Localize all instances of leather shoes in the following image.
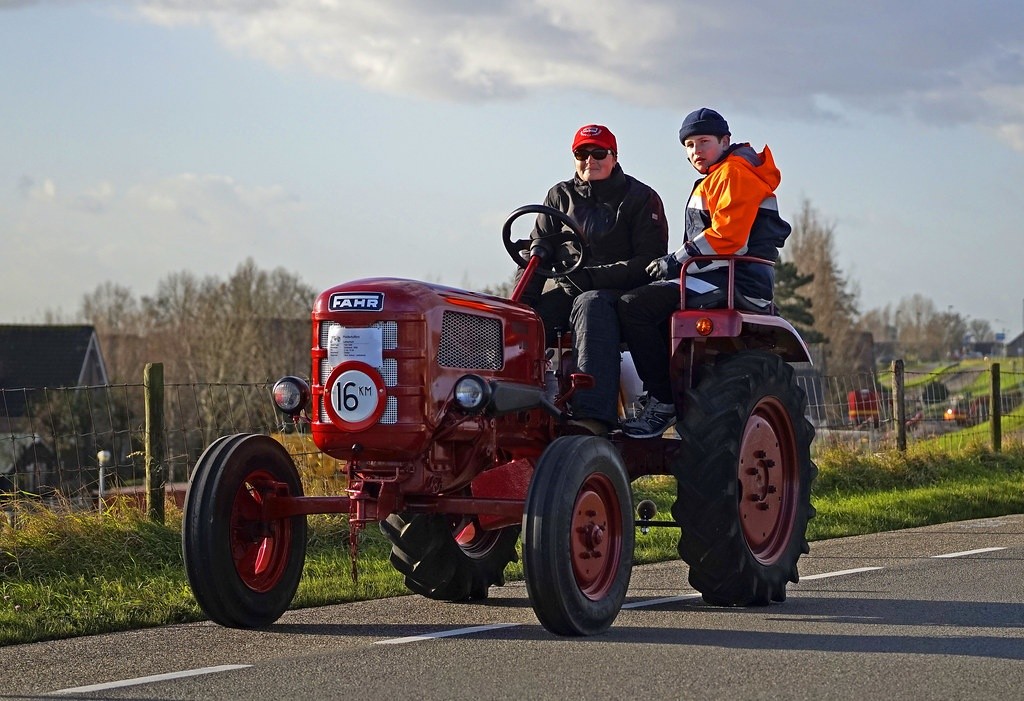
[569,418,609,438]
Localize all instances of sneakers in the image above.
[621,396,677,439]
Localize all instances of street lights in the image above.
[95,449,112,514]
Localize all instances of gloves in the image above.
[644,254,679,281]
[556,261,588,297]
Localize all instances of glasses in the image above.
[574,148,611,160]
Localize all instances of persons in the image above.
[619,107,793,438]
[516,121,668,438]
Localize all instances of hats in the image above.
[680,108,731,145]
[573,125,618,157]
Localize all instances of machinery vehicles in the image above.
[180,202,823,641]
